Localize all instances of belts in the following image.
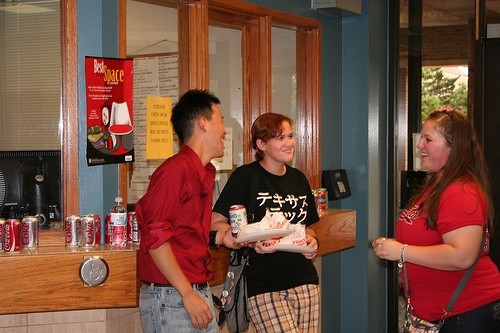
[141,282,207,291]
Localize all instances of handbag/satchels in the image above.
[403,312,440,333]
[217,250,250,333]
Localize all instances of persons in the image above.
[372,110,500,333]
[210,112,321,333]
[135,88,226,333]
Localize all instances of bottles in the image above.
[110,197,127,248]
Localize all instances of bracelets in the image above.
[311,235,319,249]
[398,244,407,268]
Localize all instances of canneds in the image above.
[316,188,329,212]
[0,216,38,252]
[64,214,101,249]
[105,212,141,246]
[229,204,248,238]
